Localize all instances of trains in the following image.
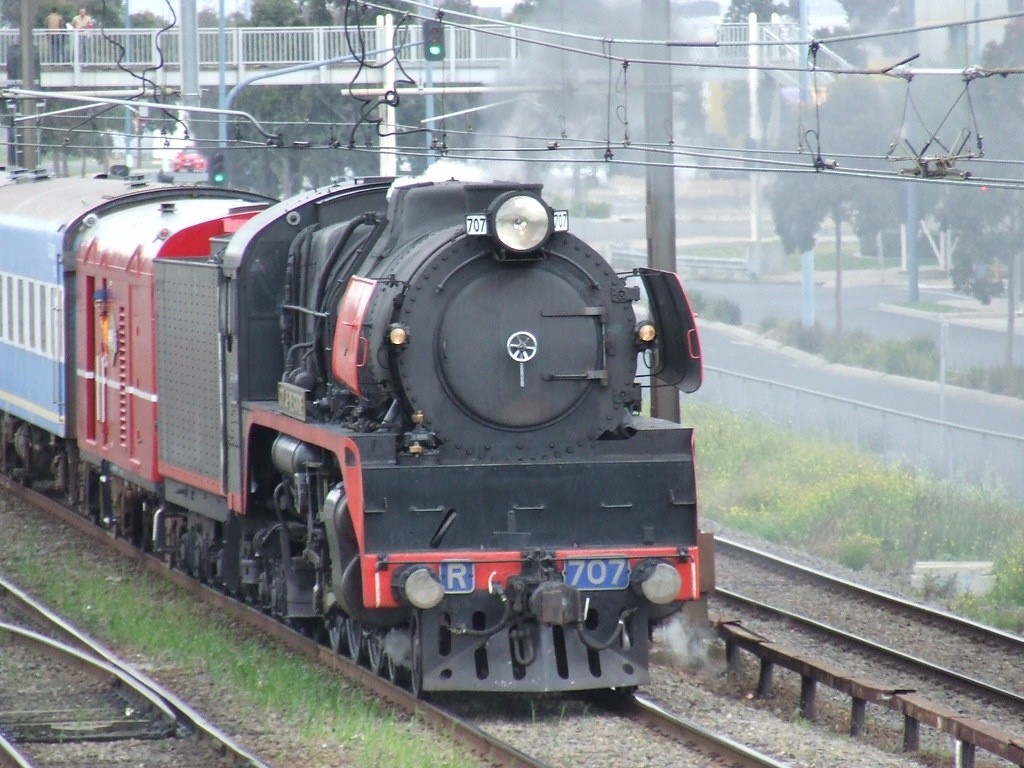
[0,163,704,716]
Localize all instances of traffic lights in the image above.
[208,150,226,186]
[421,20,446,63]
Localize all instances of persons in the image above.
[976,258,990,304]
[45,8,66,63]
[72,8,92,63]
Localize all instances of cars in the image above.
[172,144,209,173]
